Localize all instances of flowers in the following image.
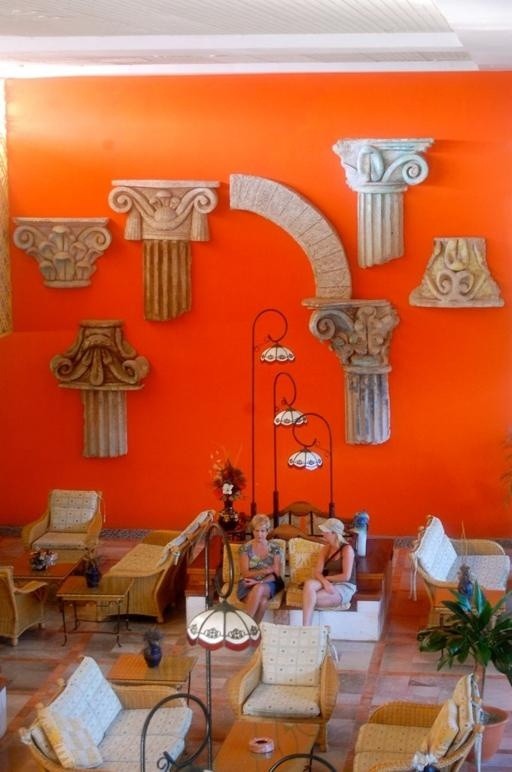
[207,445,248,501]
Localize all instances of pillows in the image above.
[35,704,104,770]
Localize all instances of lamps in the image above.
[246,308,334,526]
[186,522,263,772]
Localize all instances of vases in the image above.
[218,504,240,531]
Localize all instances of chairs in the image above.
[220,524,358,624]
[230,621,341,754]
[1,489,103,648]
[410,512,511,638]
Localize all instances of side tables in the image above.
[105,652,198,713]
[56,575,135,649]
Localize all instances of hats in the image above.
[319,517,344,535]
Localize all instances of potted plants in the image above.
[413,564,512,762]
[141,628,163,668]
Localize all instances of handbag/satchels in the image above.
[272,572,285,593]
[322,568,328,575]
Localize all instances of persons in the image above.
[302,518,358,627]
[237,514,285,624]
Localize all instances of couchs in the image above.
[97,509,216,624]
[0,652,194,772]
[352,669,486,772]
